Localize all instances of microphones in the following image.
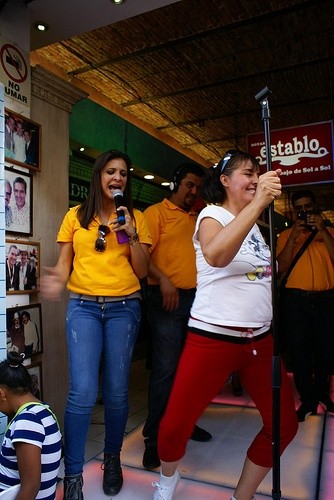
[113,189,125,225]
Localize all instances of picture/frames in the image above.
[4,104,44,423]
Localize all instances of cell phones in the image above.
[297,209,307,227]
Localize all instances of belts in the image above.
[70,292,142,302]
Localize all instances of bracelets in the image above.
[130,234,139,245]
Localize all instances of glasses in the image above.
[220,150,248,173]
[94,225,110,250]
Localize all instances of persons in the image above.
[276,189,334,423]
[0,351,61,500]
[143,163,213,470]
[11,310,38,355]
[5,177,30,232]
[5,245,37,290]
[5,115,36,165]
[39,151,153,500]
[152,150,298,500]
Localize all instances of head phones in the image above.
[169,163,203,192]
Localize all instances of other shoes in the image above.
[316,394,334,414]
[143,437,159,470]
[191,424,212,441]
[296,401,319,422]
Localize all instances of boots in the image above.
[100,452,123,495]
[63,474,84,500]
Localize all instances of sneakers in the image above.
[152,468,180,500]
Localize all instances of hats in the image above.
[12,313,20,320]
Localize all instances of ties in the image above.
[9,131,11,136]
